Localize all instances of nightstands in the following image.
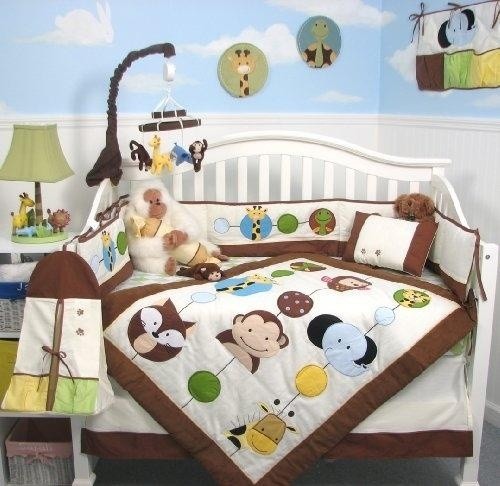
[0,233,76,264]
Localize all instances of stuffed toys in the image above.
[46,206,72,232]
[392,190,436,224]
[124,180,231,279]
[10,193,35,236]
[128,138,207,175]
[174,264,230,281]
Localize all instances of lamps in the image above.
[0,124,75,243]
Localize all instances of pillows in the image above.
[343,211,439,278]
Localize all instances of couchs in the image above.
[60,132,499,486]
